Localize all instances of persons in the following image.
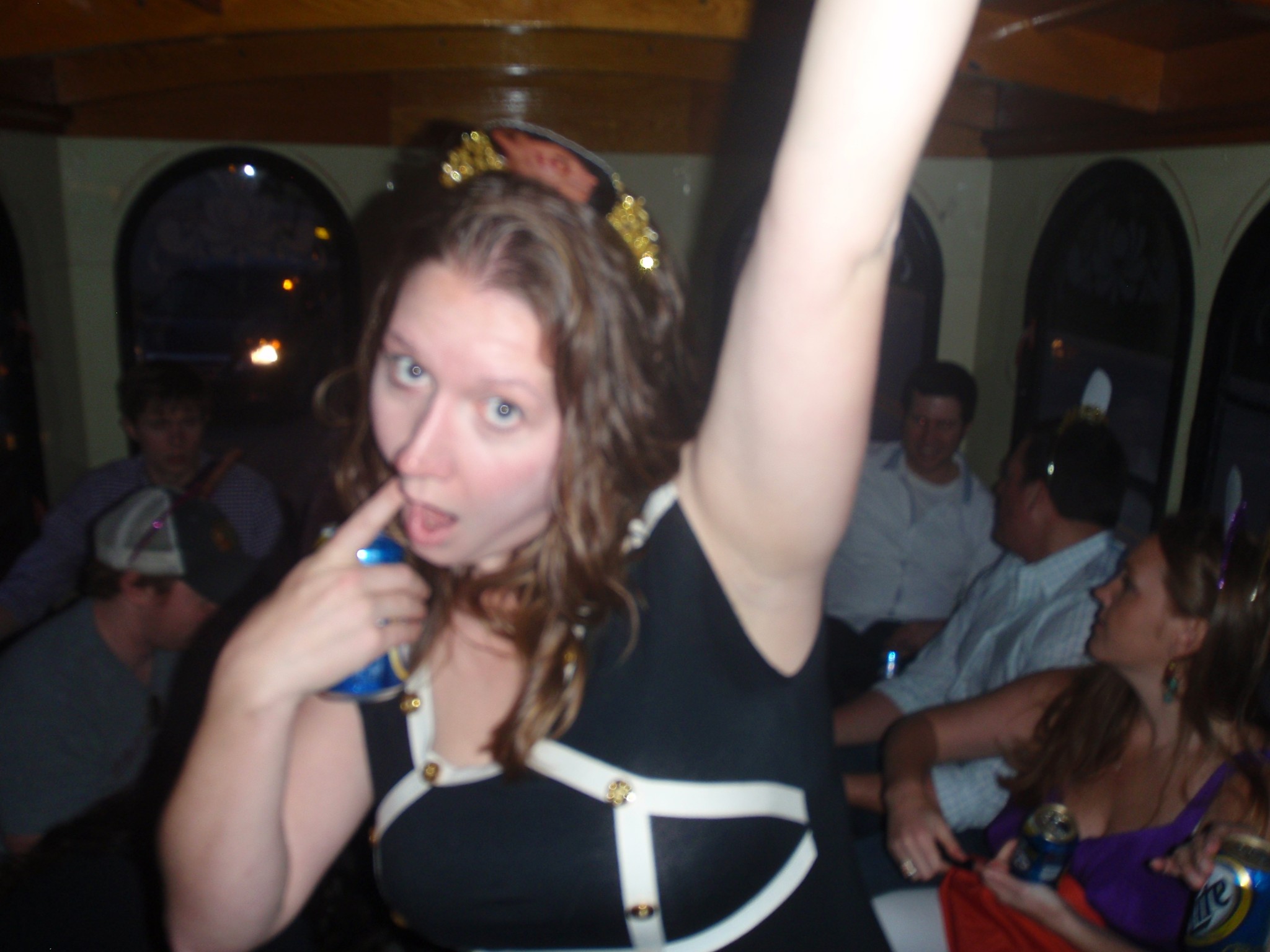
[0,363,283,952]
[825,358,1270,952]
[161,0,986,952]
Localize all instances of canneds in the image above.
[879,644,902,681]
[1178,832,1270,952]
[316,524,405,703]
[1009,803,1078,891]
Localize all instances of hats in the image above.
[92,483,255,604]
